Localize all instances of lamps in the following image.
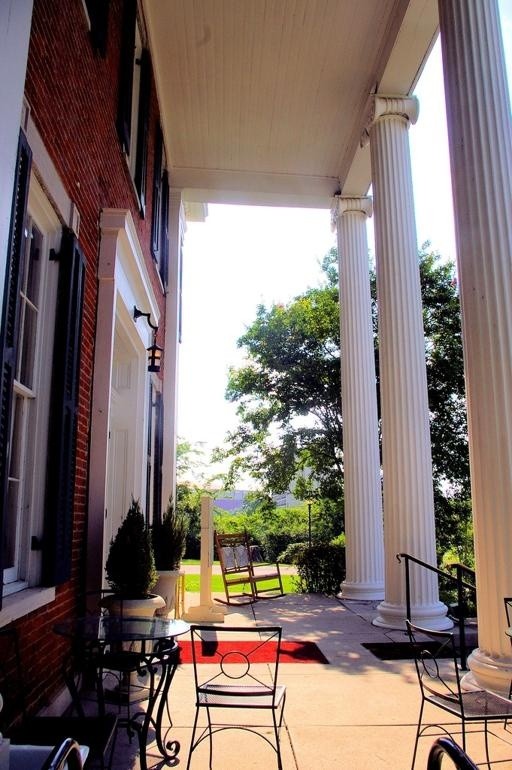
[134,305,164,372]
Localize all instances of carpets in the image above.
[360,641,459,661]
[428,634,478,657]
[168,640,330,664]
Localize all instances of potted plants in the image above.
[96,493,167,704]
[150,492,187,613]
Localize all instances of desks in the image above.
[54,615,191,770]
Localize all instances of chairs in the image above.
[80,589,174,742]
[41,737,83,770]
[213,526,286,606]
[405,619,512,770]
[0,628,118,770]
[503,597,512,730]
[427,736,479,770]
[187,625,287,770]
